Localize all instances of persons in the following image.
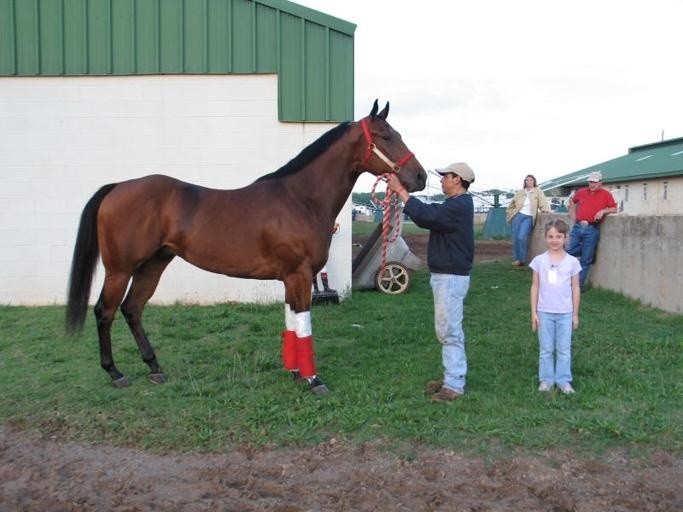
[504,174,554,267]
[561,171,618,294]
[381,161,476,404]
[526,218,583,395]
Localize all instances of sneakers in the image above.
[426,376,444,391]
[511,260,520,267]
[431,388,459,403]
[560,383,575,395]
[537,382,551,392]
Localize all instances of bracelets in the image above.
[395,187,404,198]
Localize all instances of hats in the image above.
[434,162,474,183]
[585,172,602,183]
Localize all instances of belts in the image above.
[577,220,599,227]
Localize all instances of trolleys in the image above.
[353,203,422,297]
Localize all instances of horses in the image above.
[62,99,427,397]
